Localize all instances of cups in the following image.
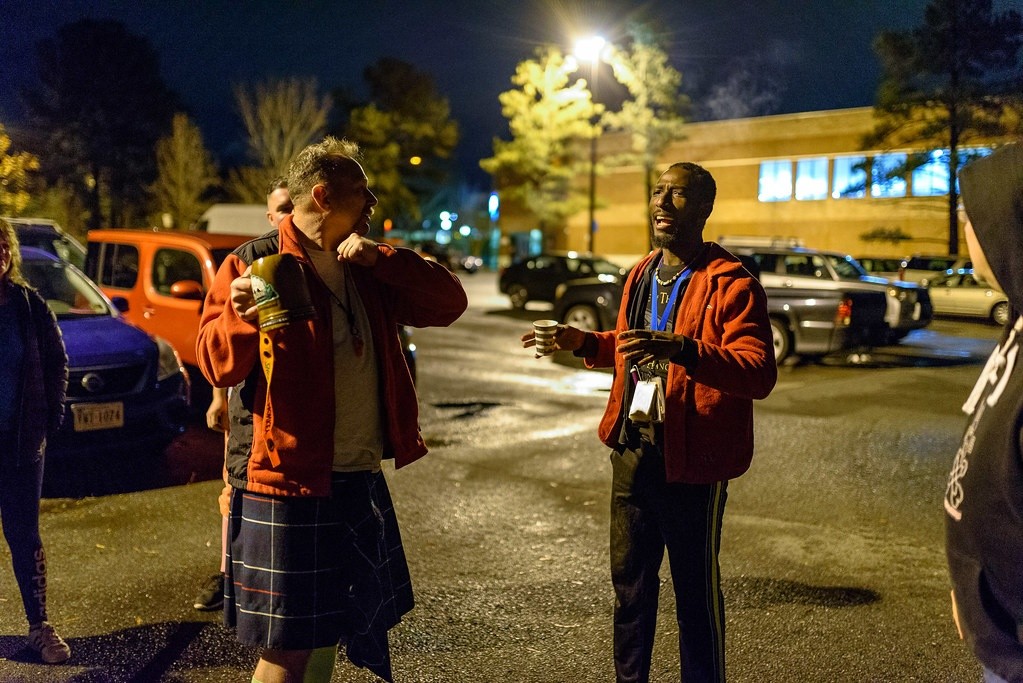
[532,319,558,356]
[250,252,316,334]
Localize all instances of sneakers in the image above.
[193,573,225,610]
[29,621,71,664]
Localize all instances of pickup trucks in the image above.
[555,243,887,365]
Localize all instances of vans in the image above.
[76,229,265,421]
[193,205,285,236]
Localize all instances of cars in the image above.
[19,248,192,473]
[920,271,1012,329]
[412,233,480,270]
[9,217,88,272]
[499,252,626,310]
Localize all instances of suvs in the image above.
[839,251,905,282]
[725,248,934,345]
[900,254,975,285]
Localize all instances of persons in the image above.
[193,138,469,683]
[0,216,71,662]
[521,161,778,683]
[944,149,1023,683]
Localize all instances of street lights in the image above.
[573,39,600,254]
